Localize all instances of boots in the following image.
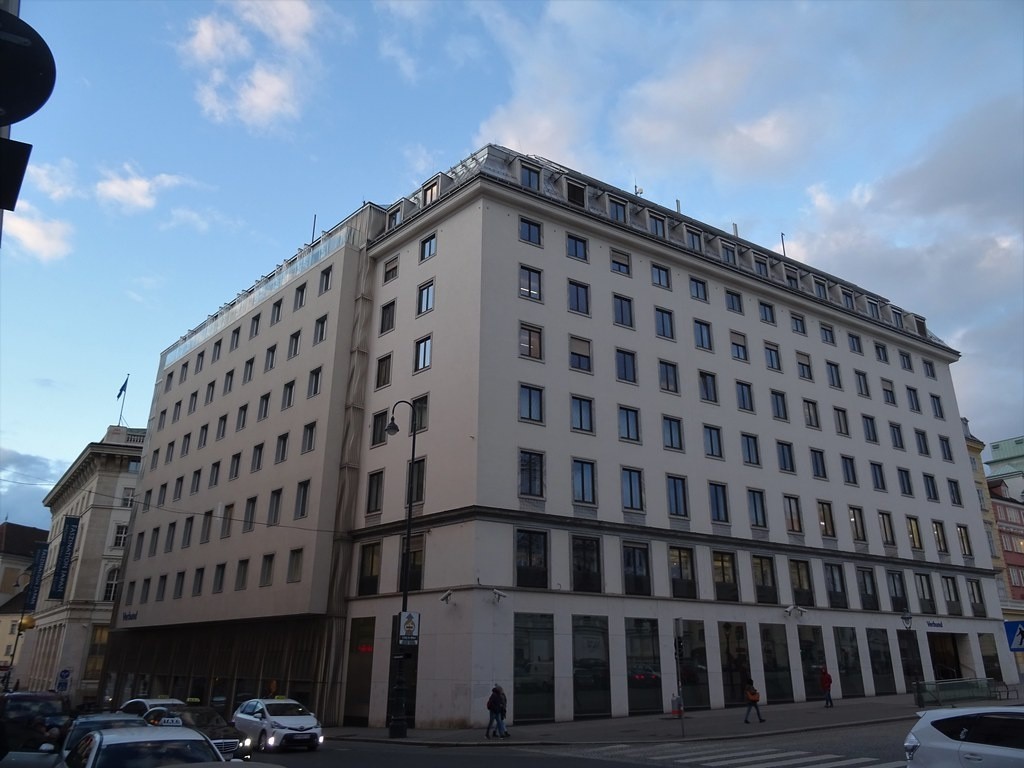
[493,731,498,737]
[504,731,510,736]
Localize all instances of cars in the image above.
[137,706,254,761]
[0,751,69,768]
[53,727,226,768]
[227,698,323,754]
[115,699,187,719]
[60,713,156,762]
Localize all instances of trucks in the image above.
[0,686,73,739]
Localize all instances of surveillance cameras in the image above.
[493,589,506,597]
[785,606,794,612]
[797,607,808,612]
[440,589,452,601]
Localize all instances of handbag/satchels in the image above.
[747,686,759,702]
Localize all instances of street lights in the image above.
[383,400,417,740]
[2,572,31,693]
[900,608,928,709]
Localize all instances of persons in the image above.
[819,666,834,708]
[485,683,511,740]
[743,679,766,725]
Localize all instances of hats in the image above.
[495,683,501,690]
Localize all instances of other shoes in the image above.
[500,735,506,738]
[760,719,766,723]
[744,720,750,724]
[485,735,490,739]
[824,704,833,708]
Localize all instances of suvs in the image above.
[902,705,1024,766]
